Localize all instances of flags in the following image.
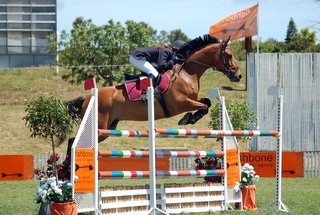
[209,3,260,43]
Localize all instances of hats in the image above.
[173,40,187,47]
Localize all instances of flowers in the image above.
[29,155,81,205]
[197,152,226,169]
[233,162,262,191]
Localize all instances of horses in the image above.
[59,33,243,179]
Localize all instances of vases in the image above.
[241,182,255,211]
[201,164,222,183]
[50,200,77,215]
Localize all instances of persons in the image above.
[128,38,190,100]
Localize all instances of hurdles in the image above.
[71,77,289,215]
